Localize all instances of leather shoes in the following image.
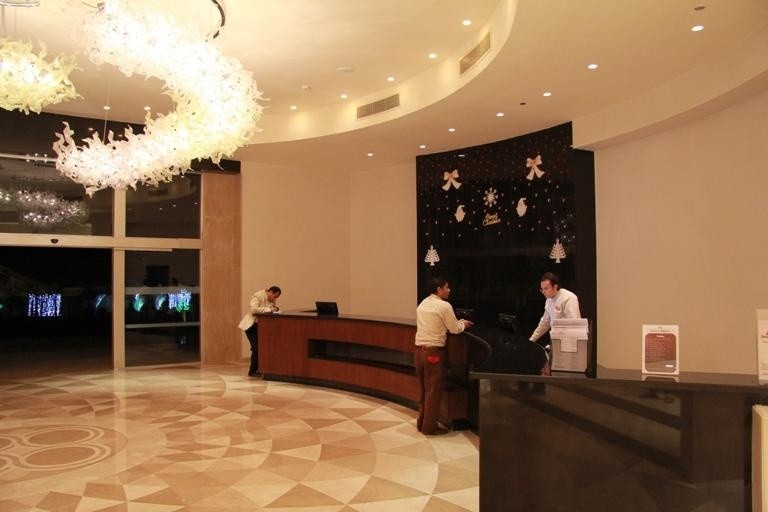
[418,427,448,435]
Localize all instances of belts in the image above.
[416,346,445,351]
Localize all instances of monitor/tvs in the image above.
[496,313,521,335]
[25,289,65,320]
[152,294,167,312]
[316,302,338,314]
[131,295,145,312]
[167,292,193,311]
[455,308,475,322]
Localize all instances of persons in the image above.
[528,271,583,344]
[414,276,475,436]
[236,286,282,379]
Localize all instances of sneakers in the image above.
[249,371,262,377]
